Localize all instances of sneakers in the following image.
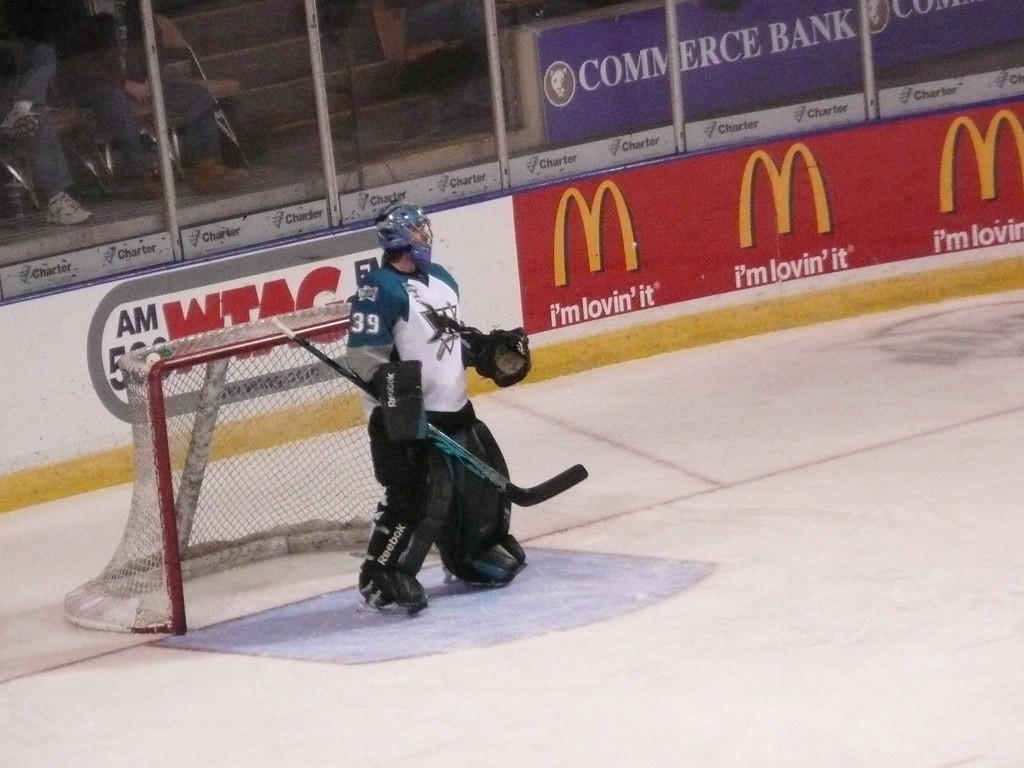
[0,106,41,142]
[44,191,92,226]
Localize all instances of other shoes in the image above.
[140,175,164,200]
[191,159,249,192]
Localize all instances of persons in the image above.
[347,201,532,615]
[0,0,249,227]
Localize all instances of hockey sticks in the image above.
[272,316,588,507]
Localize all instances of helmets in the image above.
[376,202,433,266]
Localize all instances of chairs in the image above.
[0,15,249,219]
